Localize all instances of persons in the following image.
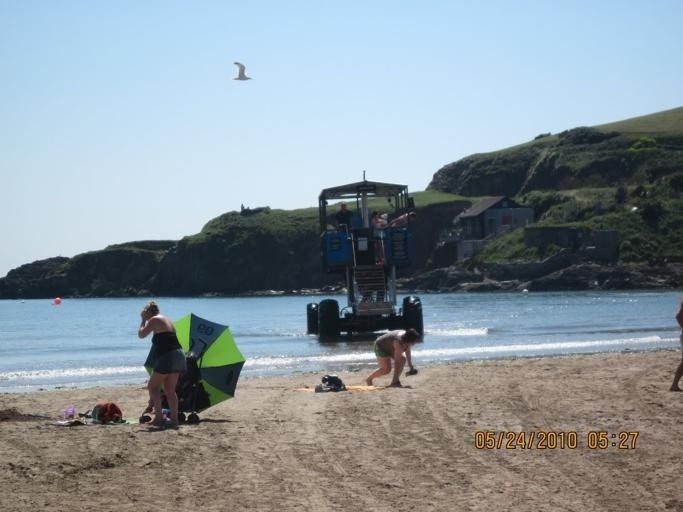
[364,328,418,388]
[332,201,416,268]
[667,302,683,391]
[138,300,186,427]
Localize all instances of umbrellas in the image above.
[143,312,243,413]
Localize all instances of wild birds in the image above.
[234,62,252,80]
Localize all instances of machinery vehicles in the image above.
[302,168,432,343]
